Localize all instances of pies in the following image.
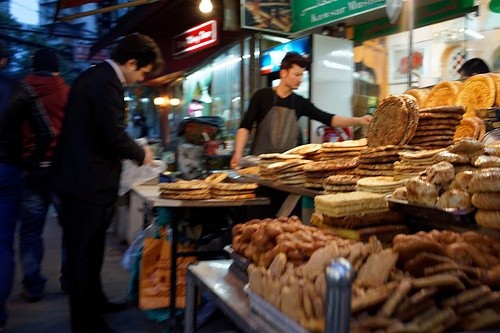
[158,72,500,202]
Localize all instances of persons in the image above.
[54,33,162,333]
[0,31,54,332]
[230,51,373,168]
[457,58,490,79]
[17,47,74,302]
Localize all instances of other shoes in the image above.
[21,288,45,303]
[0,290,137,332]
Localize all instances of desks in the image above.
[125,187,270,332]
[184,260,278,333]
[244,177,480,229]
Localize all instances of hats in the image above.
[33,49,59,72]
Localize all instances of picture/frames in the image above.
[387,46,427,85]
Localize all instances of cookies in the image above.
[231,139,500,333]
